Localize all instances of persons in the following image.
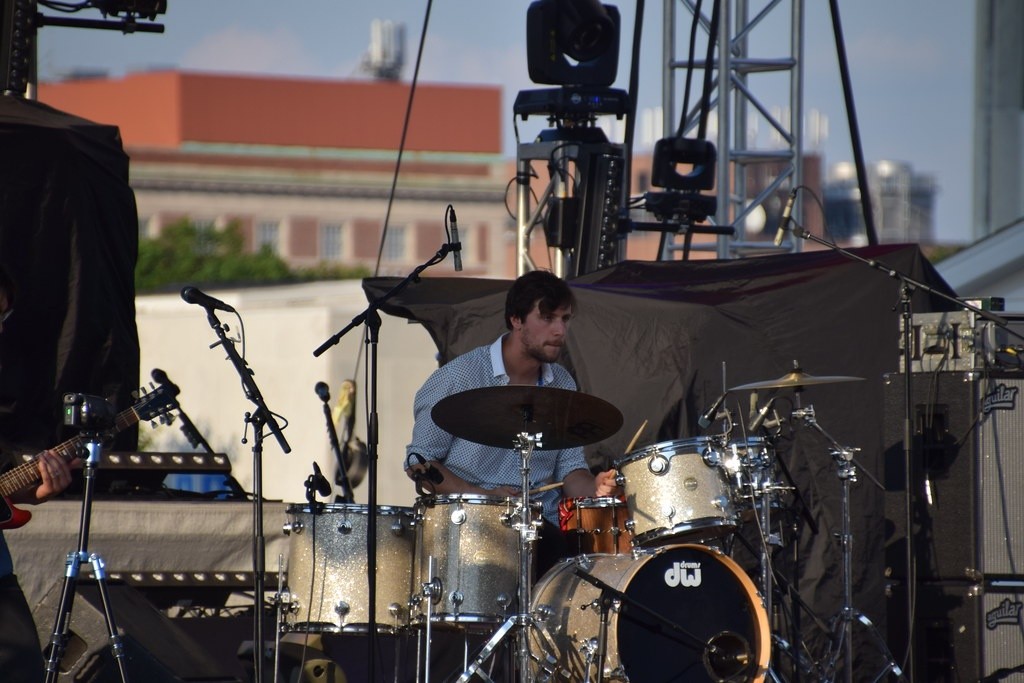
[404,270,623,570]
[0,248,82,682]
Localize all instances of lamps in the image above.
[512,0,637,119]
[646,134,721,221]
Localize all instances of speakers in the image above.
[887,578,1024,683]
[31,576,243,683]
[881,372,1024,581]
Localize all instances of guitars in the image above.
[0,378,180,530]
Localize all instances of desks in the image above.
[3,499,298,683]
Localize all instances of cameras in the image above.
[62,392,116,430]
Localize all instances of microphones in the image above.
[152,369,180,395]
[698,392,728,429]
[415,453,445,485]
[449,209,463,271]
[711,651,754,668]
[181,286,235,313]
[315,382,331,402]
[313,462,332,496]
[774,188,797,246]
[747,398,774,432]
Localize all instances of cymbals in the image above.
[728,373,868,391]
[430,386,624,451]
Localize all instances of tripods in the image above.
[45,430,132,683]
[789,384,910,683]
[456,421,578,683]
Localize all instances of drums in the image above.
[558,495,634,558]
[612,436,740,550]
[720,436,787,523]
[408,491,543,635]
[276,501,419,636]
[522,545,772,683]
